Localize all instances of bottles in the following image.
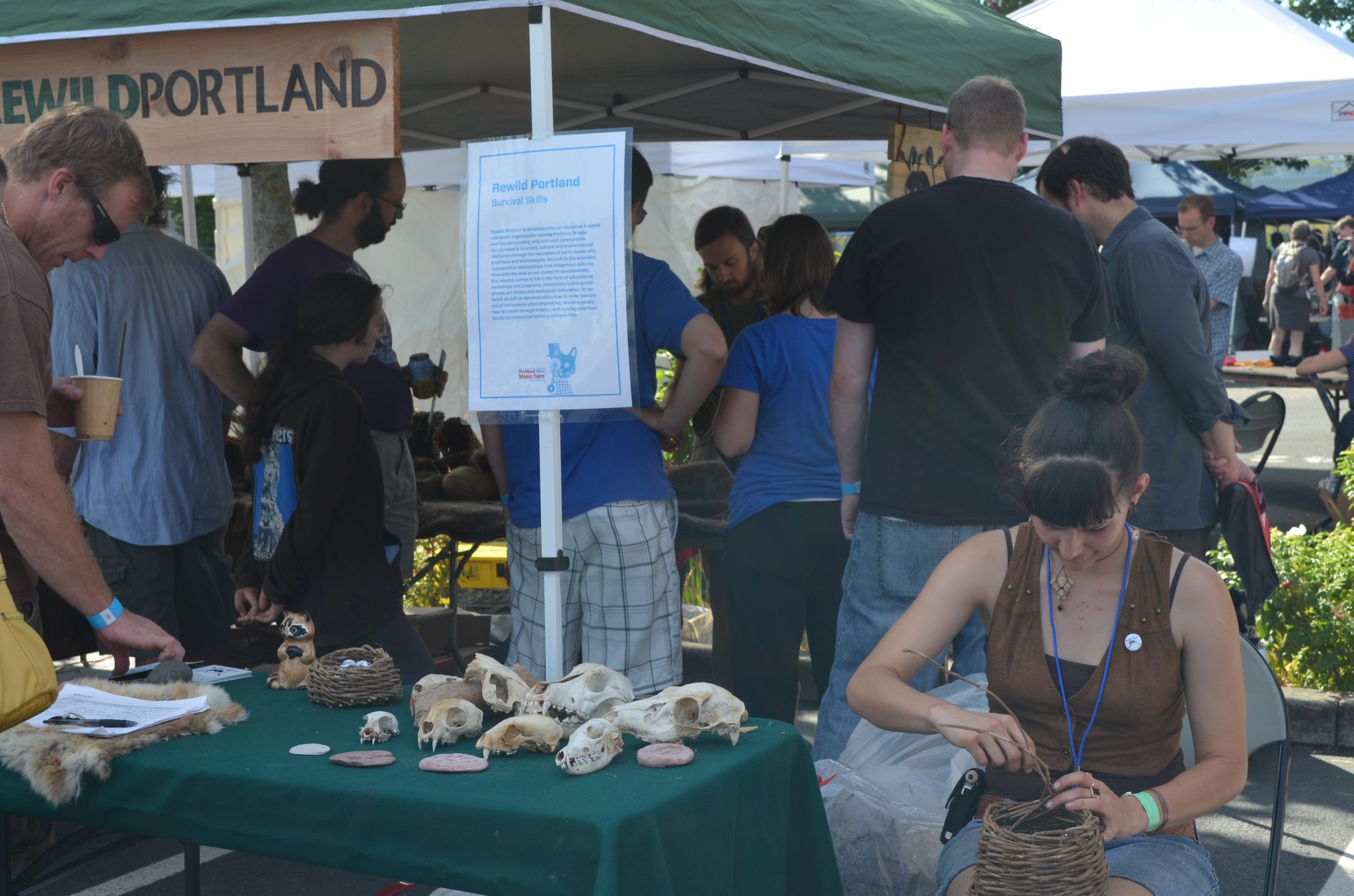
[408,353,436,400]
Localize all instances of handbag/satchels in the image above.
[0,556,59,733]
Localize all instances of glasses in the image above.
[755,226,768,253]
[367,186,408,218]
[1174,221,1207,234]
[74,173,121,247]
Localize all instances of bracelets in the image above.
[1121,789,1169,831]
[85,596,123,628]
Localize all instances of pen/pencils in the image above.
[43,717,137,729]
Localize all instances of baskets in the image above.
[306,647,403,706]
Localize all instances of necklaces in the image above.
[1050,527,1125,611]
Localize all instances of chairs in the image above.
[1179,632,1290,896]
[1233,391,1285,475]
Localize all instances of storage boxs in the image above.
[457,541,510,589]
[404,605,499,676]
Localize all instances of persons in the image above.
[477,145,729,700]
[1,107,184,675]
[1177,194,1243,372]
[845,343,1248,896]
[815,73,1107,763]
[190,155,448,577]
[709,212,849,729]
[1263,218,1354,499]
[1035,135,1257,555]
[47,168,234,664]
[661,207,772,610]
[235,274,439,681]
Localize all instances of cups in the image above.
[70,375,123,441]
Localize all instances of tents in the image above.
[1011,161,1262,237]
[0,0,1066,685]
[159,0,1354,233]
[1229,167,1354,358]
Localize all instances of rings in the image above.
[1085,772,1094,788]
[1089,788,1100,798]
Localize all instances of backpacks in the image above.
[1274,242,1308,288]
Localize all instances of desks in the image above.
[403,500,728,672]
[0,671,844,896]
[1222,365,1349,432]
